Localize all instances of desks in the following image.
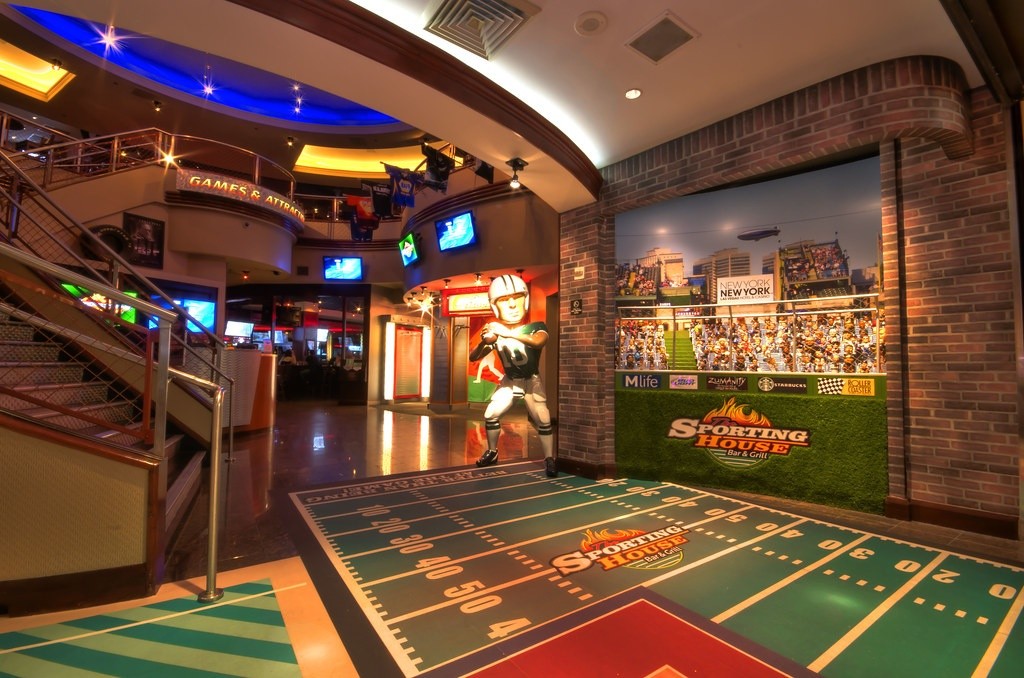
[16,140,48,156]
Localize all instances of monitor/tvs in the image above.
[323,255,364,282]
[434,209,479,253]
[224,321,255,344]
[397,231,421,268]
[307,340,314,350]
[268,331,283,344]
[147,294,217,335]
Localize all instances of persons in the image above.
[469,275,558,477]
[138,223,152,240]
[614,244,886,373]
[279,347,321,384]
